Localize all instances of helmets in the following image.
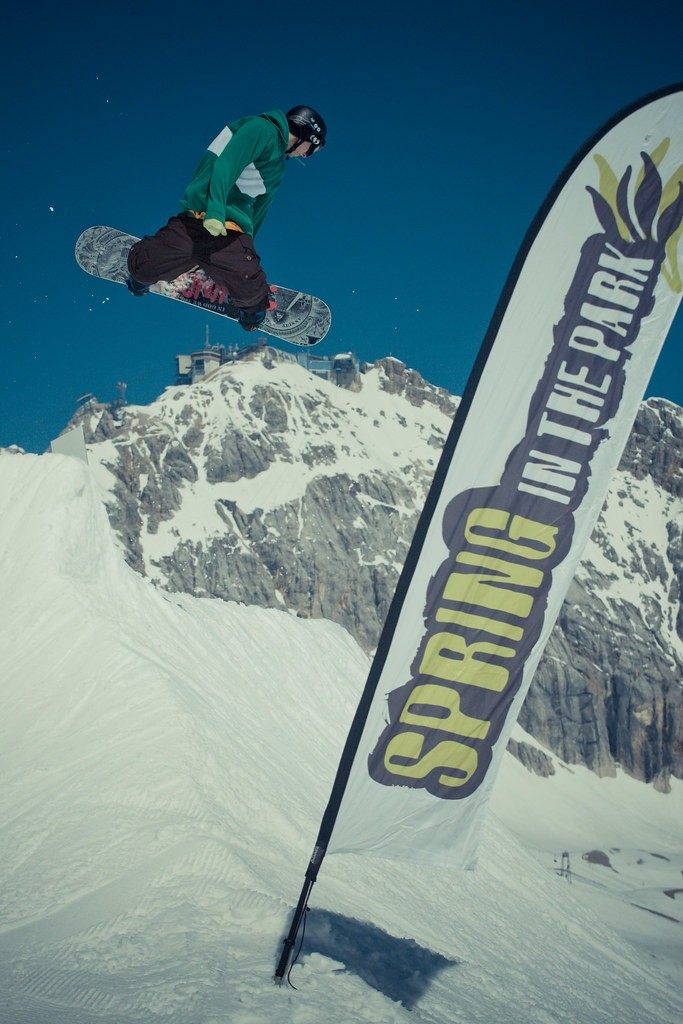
[285,105,325,146]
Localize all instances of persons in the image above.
[128,105,327,330]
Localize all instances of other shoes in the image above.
[128,274,149,296]
[239,310,266,330]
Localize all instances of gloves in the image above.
[204,219,228,238]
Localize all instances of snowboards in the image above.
[74,225,333,346]
